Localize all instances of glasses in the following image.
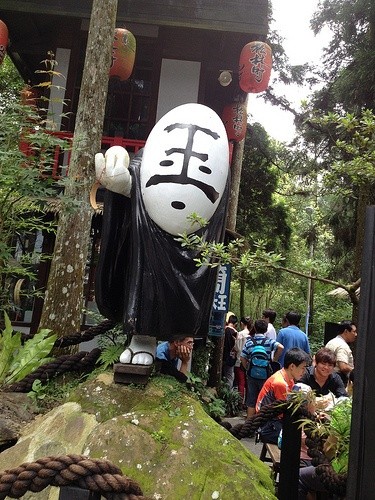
[179,340,194,347]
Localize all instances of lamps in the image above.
[218,70,233,86]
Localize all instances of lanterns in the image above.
[221,102,248,144]
[0,20,9,65]
[109,27,137,83]
[18,84,40,123]
[238,40,273,93]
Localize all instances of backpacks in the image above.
[256,420,282,445]
[246,337,273,383]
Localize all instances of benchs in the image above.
[259,438,311,486]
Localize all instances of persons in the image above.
[298,369,354,500]
[324,321,358,388]
[240,319,284,418]
[221,309,346,450]
[155,337,194,384]
[96,101,231,386]
